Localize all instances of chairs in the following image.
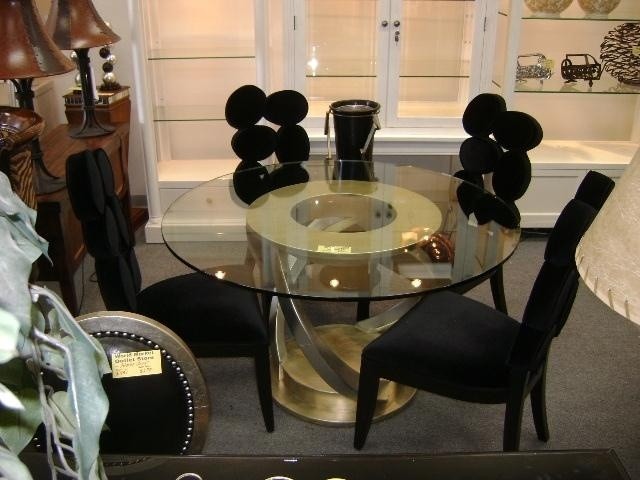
[74,311,209,454]
[225,84,370,320]
[446,93,543,314]
[354,170,616,450]
[66,148,274,433]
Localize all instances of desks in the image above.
[63,100,149,231]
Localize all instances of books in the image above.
[63,85,130,106]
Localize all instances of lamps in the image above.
[0,0,77,196]
[45,0,122,138]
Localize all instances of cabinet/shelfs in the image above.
[127,0,498,244]
[492,0,640,228]
[36,121,136,317]
[515,170,626,229]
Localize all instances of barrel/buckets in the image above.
[325,98,383,161]
[324,159,380,195]
